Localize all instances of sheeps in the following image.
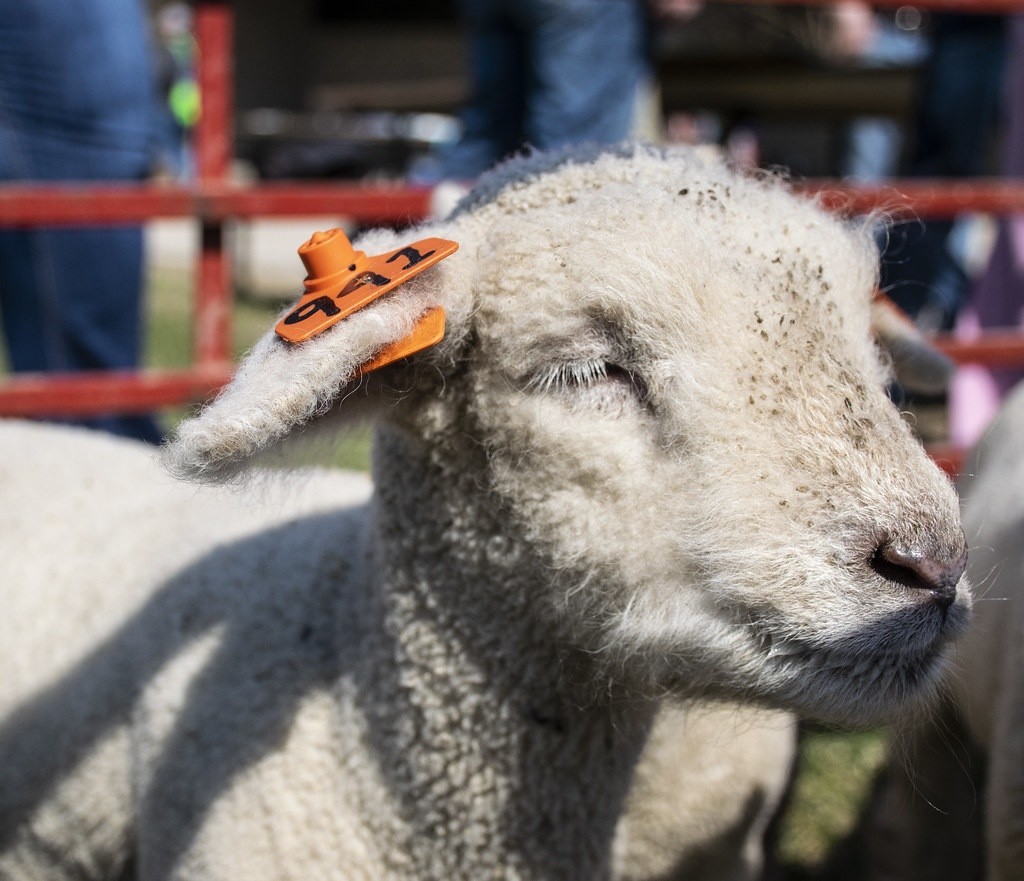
[0,143,1012,881]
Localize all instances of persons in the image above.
[158,0,1024,446]
[0,0,175,447]
